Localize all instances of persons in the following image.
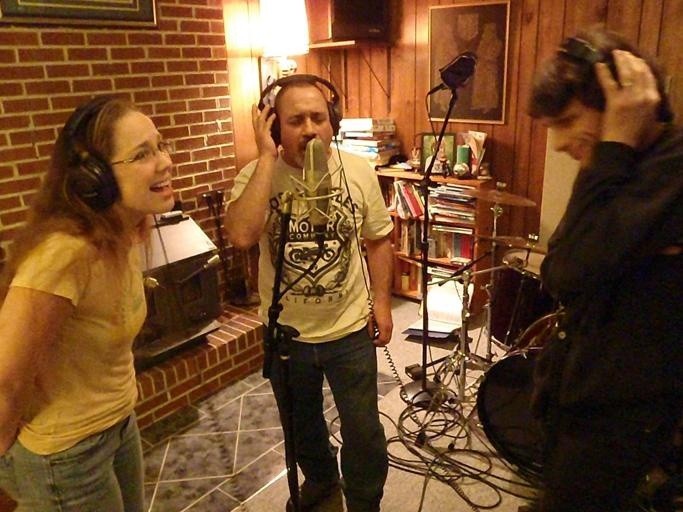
[477,23,683,512]
[0,95,175,512]
[223,74,395,512]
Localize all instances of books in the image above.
[330,117,405,173]
[392,181,476,334]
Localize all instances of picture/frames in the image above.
[426,1,511,127]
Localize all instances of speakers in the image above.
[308,0,385,43]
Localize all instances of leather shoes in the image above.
[286,472,340,512]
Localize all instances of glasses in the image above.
[111,138,177,167]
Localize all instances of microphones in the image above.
[304,139,332,247]
[438,51,478,90]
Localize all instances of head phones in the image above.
[61,96,118,209]
[558,36,622,94]
[258,73,343,147]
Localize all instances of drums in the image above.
[477,312,564,485]
[484,247,560,351]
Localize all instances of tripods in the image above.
[412,205,501,453]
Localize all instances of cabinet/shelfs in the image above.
[360,170,497,319]
